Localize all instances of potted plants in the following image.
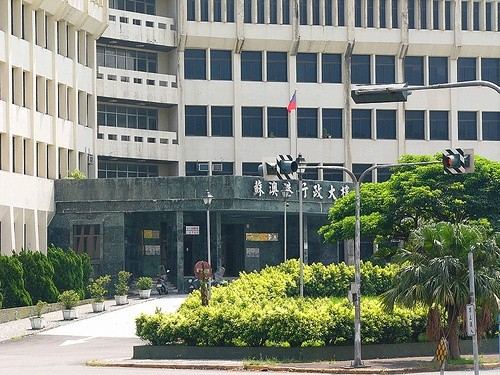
[85,275,111,312]
[136,277,154,298]
[58,290,80,319]
[30,300,47,329]
[114,270,132,305]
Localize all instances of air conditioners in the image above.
[88,156,94,165]
[196,164,209,171]
[213,164,223,172]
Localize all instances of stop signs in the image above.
[193,261,212,280]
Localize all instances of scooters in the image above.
[156,269,170,295]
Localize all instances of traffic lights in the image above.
[443,148,466,175]
[276,155,298,181]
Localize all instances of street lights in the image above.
[203,188,214,300]
